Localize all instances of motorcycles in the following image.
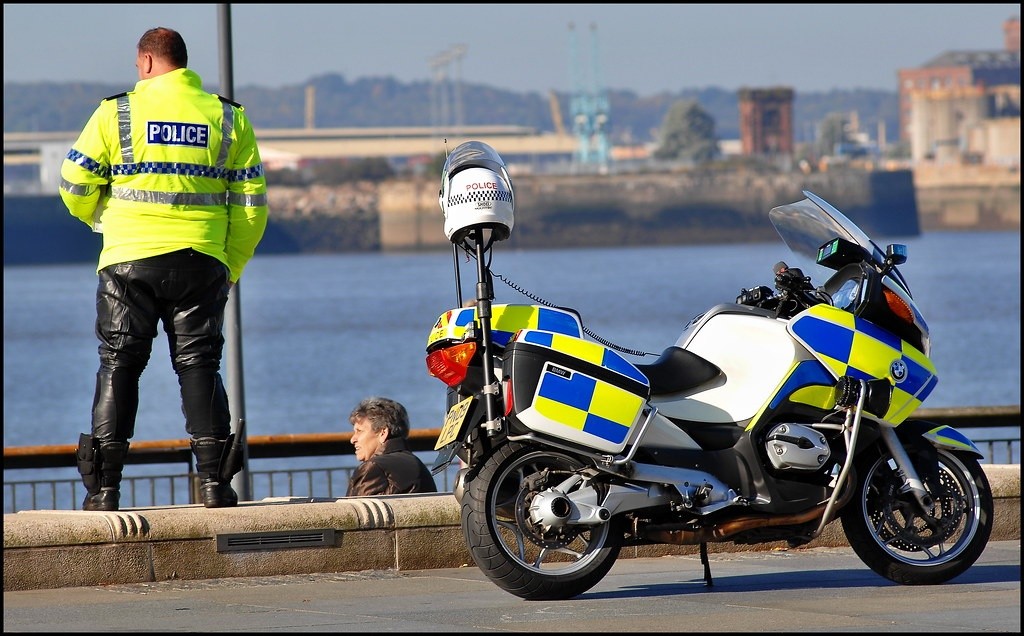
[424,139,995,604]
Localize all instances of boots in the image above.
[189,434,238,508]
[75,433,130,511]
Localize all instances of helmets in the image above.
[438,141,514,198]
[439,168,515,240]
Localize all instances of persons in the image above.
[346,397,437,499]
[57,25,270,511]
